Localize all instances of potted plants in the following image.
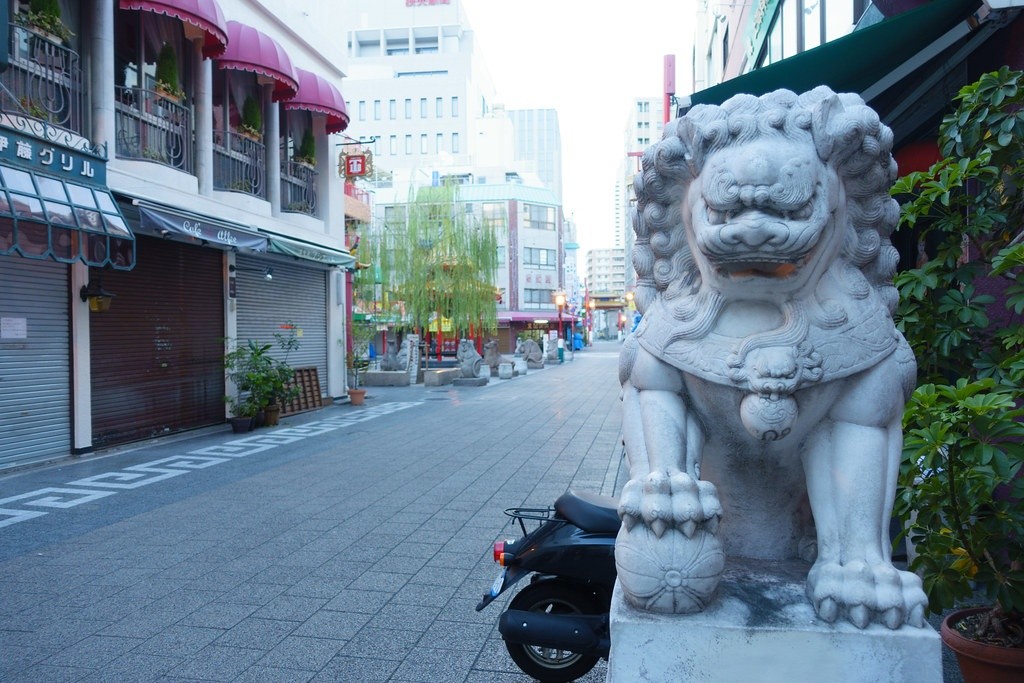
[237,94,263,142]
[898,380,1024,683]
[9,0,76,46]
[295,128,317,169]
[337,318,378,406]
[213,318,301,433]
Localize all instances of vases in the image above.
[151,42,186,104]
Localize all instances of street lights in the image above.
[556,295,566,363]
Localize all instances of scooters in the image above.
[472,489,622,683]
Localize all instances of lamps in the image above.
[229,264,273,280]
[79,277,117,312]
